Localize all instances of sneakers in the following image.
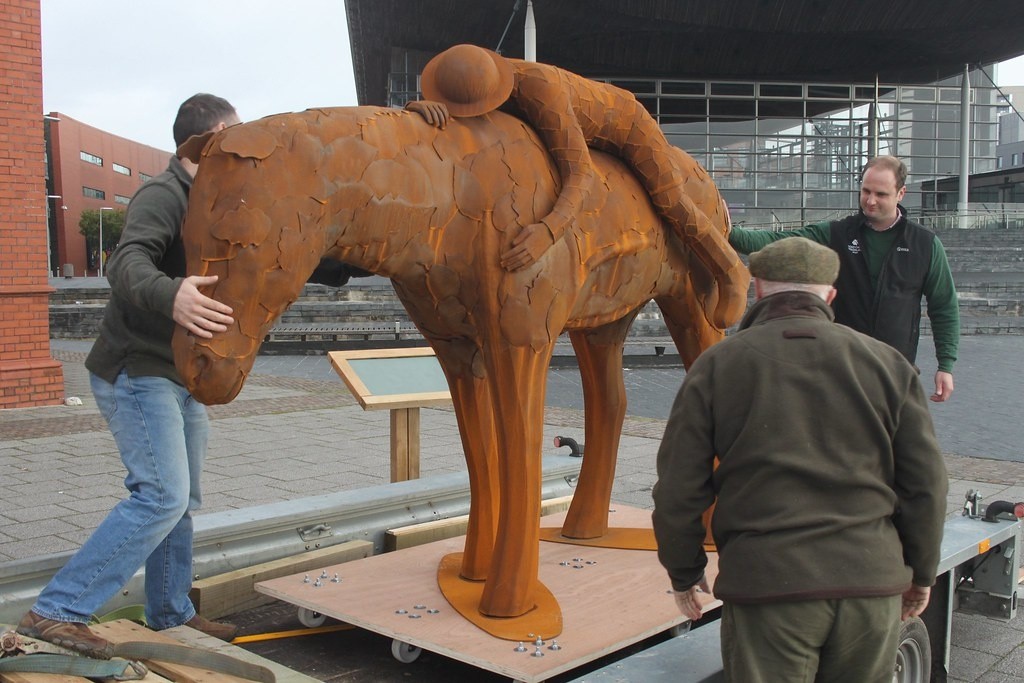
[186,613,239,644]
[16,609,114,661]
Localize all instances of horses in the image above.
[169,107,731,618]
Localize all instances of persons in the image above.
[652,237,949,682]
[18,93,242,660]
[729,156,961,401]
[406,44,751,330]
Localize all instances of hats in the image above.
[420,43,513,117]
[749,237,840,286]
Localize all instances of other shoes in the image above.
[713,257,751,330]
